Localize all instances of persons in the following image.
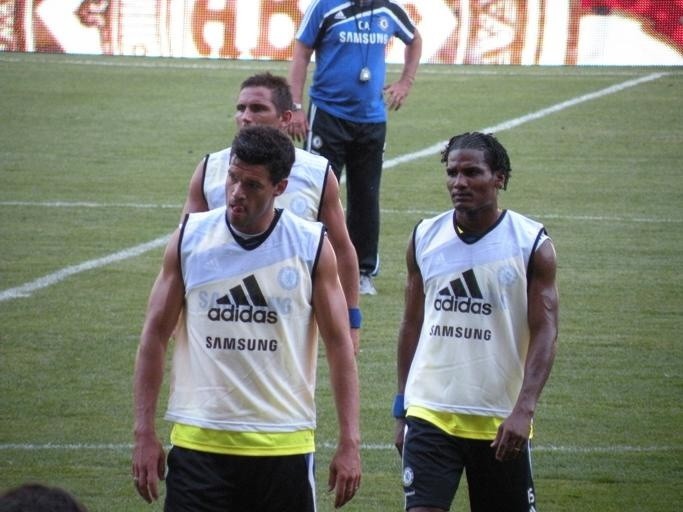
[393,131,559,512]
[287,0,422,297]
[131,125,362,512]
[0,483,89,512]
[179,74,361,355]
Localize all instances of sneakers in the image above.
[359,275,378,296]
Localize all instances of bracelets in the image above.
[393,394,405,416]
